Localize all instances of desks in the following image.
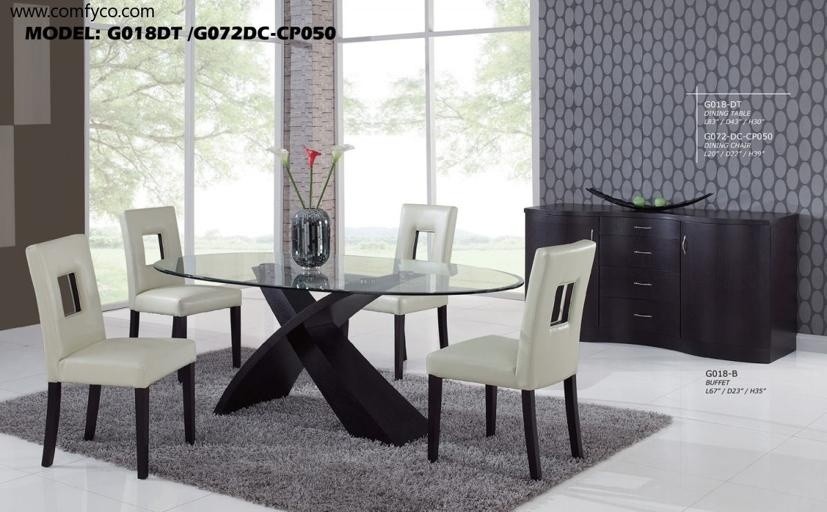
[154,252,524,446]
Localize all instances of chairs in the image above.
[121,207,242,381]
[427,238,596,480]
[25,233,195,479]
[363,205,457,379]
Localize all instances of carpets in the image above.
[0,346,673,512]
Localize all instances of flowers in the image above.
[268,144,354,208]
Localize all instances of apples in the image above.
[632,195,645,208]
[655,198,666,207]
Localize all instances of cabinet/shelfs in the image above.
[524,205,797,364]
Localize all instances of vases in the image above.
[292,271,330,289]
[291,208,330,271]
